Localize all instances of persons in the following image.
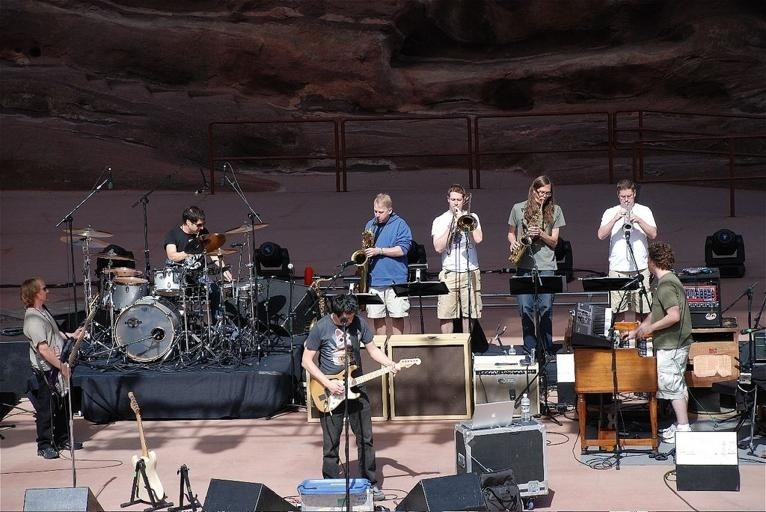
[19,278,88,459]
[597,179,659,342]
[506,176,567,364]
[163,204,234,325]
[431,184,484,360]
[363,192,413,336]
[298,295,402,504]
[619,242,696,444]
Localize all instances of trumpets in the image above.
[622,202,633,231]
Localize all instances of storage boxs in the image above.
[298,477,374,511]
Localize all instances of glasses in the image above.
[193,223,206,228]
[536,190,552,197]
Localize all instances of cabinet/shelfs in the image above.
[573,345,659,457]
[683,318,741,389]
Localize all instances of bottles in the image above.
[521,393,531,424]
[304,263,313,286]
[508,345,516,354]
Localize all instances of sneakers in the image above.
[370,483,384,501]
[53,442,82,449]
[657,425,692,443]
[38,445,59,459]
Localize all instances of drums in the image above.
[114,296,182,363]
[114,285,147,311]
[154,270,185,296]
[224,281,261,301]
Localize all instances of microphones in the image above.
[341,259,357,265]
[625,231,631,239]
[194,188,206,194]
[619,274,644,289]
[223,164,227,174]
[740,327,764,334]
[106,167,115,191]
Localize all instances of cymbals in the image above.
[226,221,268,234]
[103,267,143,275]
[113,276,148,284]
[186,234,238,260]
[63,227,113,238]
[91,252,137,261]
[61,235,110,249]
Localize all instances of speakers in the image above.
[474,353,541,418]
[674,430,740,491]
[201,478,298,512]
[22,487,103,512]
[395,472,488,512]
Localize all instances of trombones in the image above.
[445,193,478,255]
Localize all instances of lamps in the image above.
[705,227,746,279]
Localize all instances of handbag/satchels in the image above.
[25,375,39,394]
[480,468,522,512]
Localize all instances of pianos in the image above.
[571,302,614,348]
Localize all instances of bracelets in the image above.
[379,246,386,256]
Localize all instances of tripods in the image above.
[264,267,307,420]
[80,289,280,367]
[531,368,564,426]
[583,343,650,470]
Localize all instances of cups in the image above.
[531,349,536,364]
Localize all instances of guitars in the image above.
[127,391,164,503]
[309,359,421,413]
[49,306,98,396]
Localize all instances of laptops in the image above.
[463,400,515,429]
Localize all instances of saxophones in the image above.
[508,199,545,266]
[351,217,378,293]
